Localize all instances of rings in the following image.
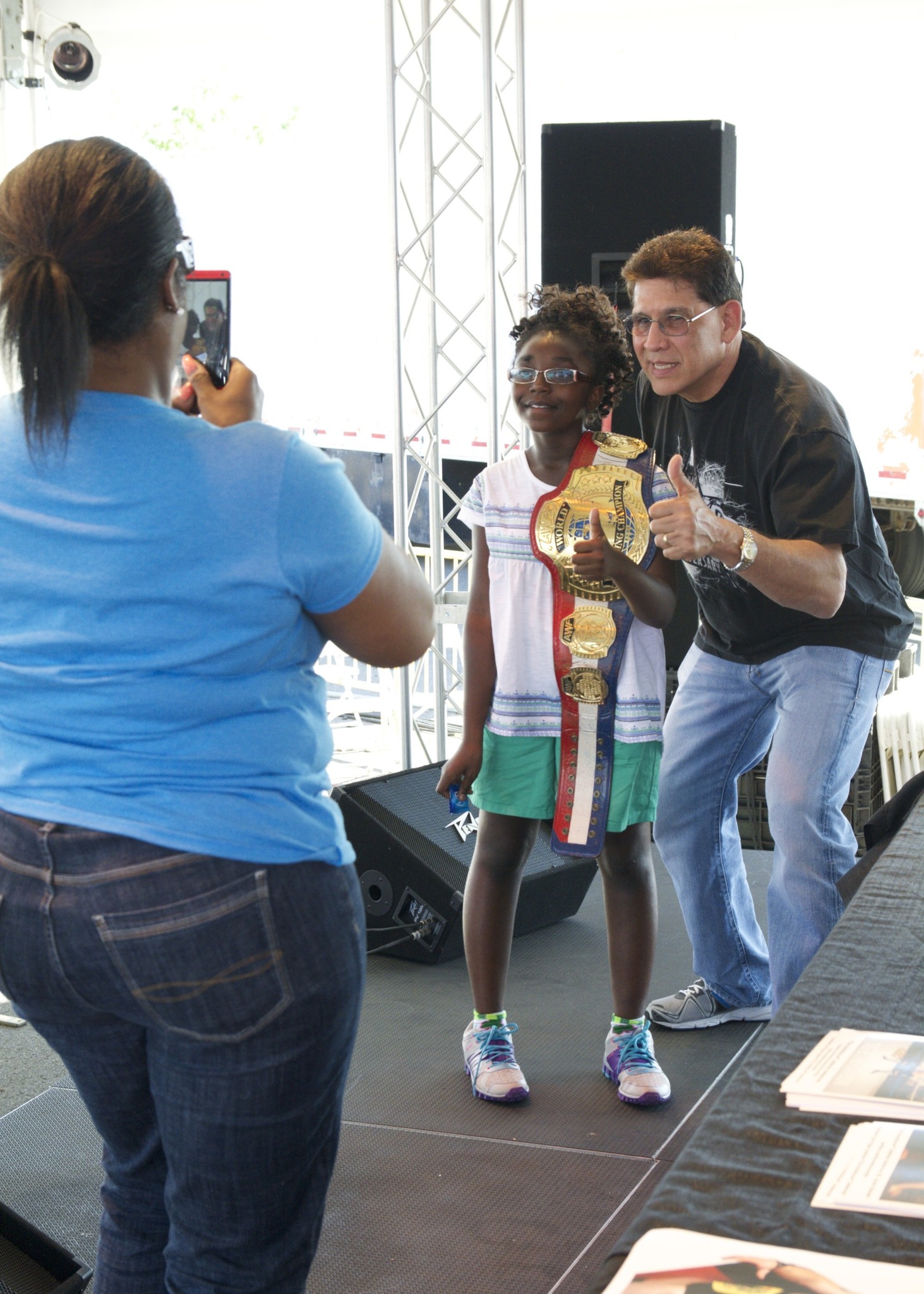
[663,534,673,546]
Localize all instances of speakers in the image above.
[543,121,736,439]
[328,760,599,966]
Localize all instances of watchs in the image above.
[723,526,758,573]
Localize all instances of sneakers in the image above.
[462,1020,530,1103]
[645,978,773,1030]
[602,1021,672,1106]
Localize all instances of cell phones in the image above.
[169,271,230,417]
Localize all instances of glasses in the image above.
[508,367,599,385]
[173,235,195,274]
[622,306,719,336]
[206,312,219,319]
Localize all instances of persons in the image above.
[0,137,436,1294]
[610,231,915,1031]
[173,299,225,381]
[436,284,675,1104]
